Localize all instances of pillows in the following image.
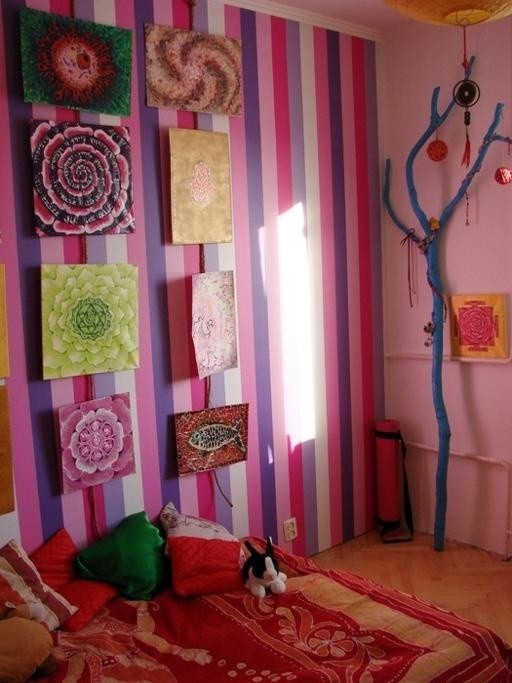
[0,538,80,633]
[76,512,171,601]
[33,528,116,634]
[0,617,53,683]
[160,500,250,598]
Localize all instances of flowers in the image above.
[40,264,140,380]
[59,391,136,496]
[27,121,137,238]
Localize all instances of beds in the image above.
[0,536,510,679]
